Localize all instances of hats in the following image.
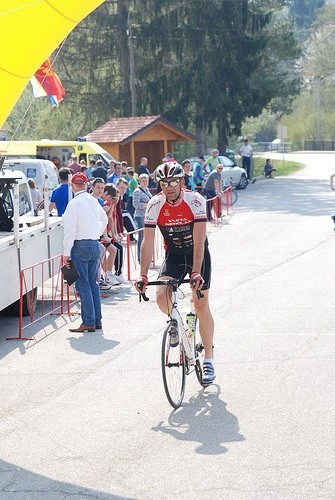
[71,172,88,184]
[167,153,173,157]
[199,156,206,162]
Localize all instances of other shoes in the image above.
[115,274,129,284]
[103,274,119,285]
[97,279,111,290]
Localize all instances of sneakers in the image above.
[202,361,216,384]
[167,319,179,347]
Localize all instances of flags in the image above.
[30,57,66,107]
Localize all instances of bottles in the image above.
[184,324,193,341]
[187,311,196,336]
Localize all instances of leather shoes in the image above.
[68,324,95,332]
[94,325,102,329]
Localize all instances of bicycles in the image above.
[137,279,214,409]
[220,177,238,206]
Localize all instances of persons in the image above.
[132,173,154,264]
[239,139,253,178]
[26,149,226,290]
[133,160,217,384]
[19,196,27,216]
[61,171,108,332]
[263,159,276,178]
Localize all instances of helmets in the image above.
[154,161,185,182]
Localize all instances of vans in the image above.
[0,140,138,233]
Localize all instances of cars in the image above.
[181,155,248,189]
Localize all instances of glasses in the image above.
[159,178,182,188]
[218,168,223,170]
